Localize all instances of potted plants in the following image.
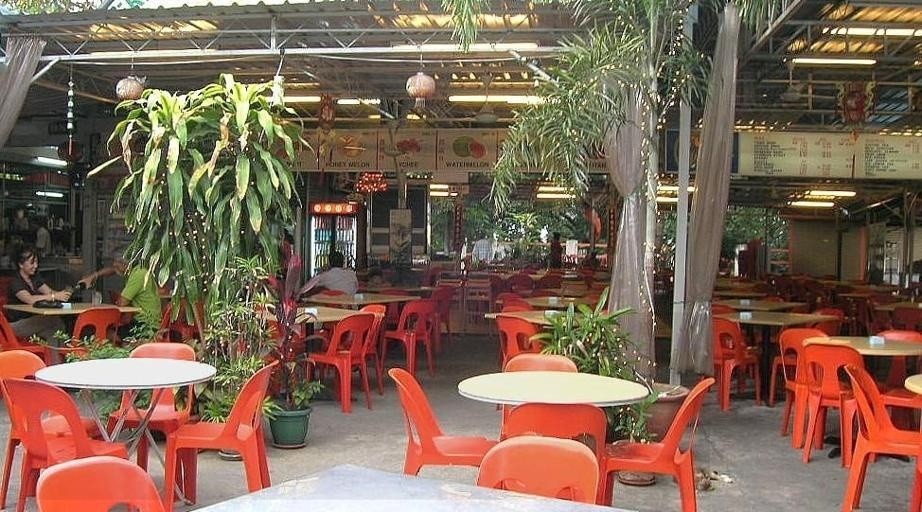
[611,390,658,486]
[264,254,325,450]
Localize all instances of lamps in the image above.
[782,22,921,67]
[387,37,543,54]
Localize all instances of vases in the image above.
[641,378,691,444]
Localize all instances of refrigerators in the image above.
[307,202,367,280]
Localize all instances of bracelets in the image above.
[52,294,56,302]
[92,271,99,281]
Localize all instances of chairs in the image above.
[107,341,196,504]
[599,378,716,510]
[35,455,164,511]
[388,368,503,491]
[164,359,280,512]
[802,343,894,471]
[778,328,846,435]
[867,386,921,464]
[712,316,762,412]
[0,350,107,510]
[843,363,921,490]
[0,245,918,368]
[475,435,597,504]
[768,309,843,407]
[503,354,578,375]
[877,330,922,383]
[305,313,374,414]
[2,376,129,511]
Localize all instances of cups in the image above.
[66,288,74,293]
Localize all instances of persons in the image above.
[4,246,74,347]
[315,250,360,310]
[546,230,563,269]
[472,232,492,264]
[0,201,71,263]
[364,265,392,287]
[380,264,421,288]
[77,246,163,339]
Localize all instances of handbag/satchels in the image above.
[33,300,61,308]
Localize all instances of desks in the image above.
[32,358,219,484]
[457,371,650,408]
[905,373,922,396]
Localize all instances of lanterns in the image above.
[116,76,143,110]
[406,71,437,109]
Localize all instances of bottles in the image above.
[76,281,97,291]
[314,214,354,267]
